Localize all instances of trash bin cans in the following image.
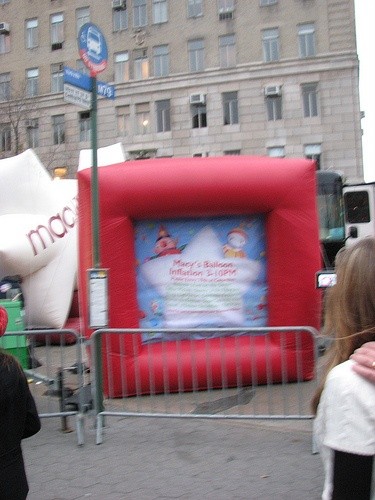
[1,298,28,371]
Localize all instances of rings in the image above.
[371,361,375,369]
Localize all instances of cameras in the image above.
[315,271,338,288]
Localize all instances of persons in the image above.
[309,240,375,495]
[350,340,375,385]
